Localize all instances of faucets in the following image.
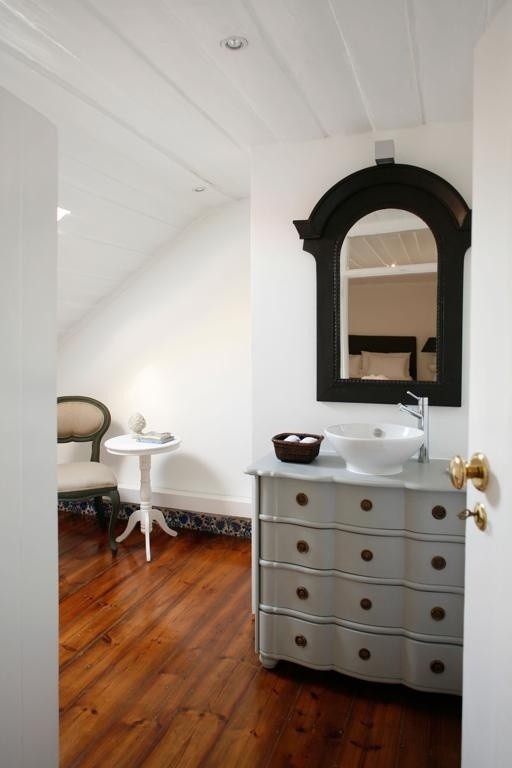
[398,391,430,463]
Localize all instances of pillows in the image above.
[347,348,417,382]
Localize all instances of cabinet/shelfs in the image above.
[254,477,463,696]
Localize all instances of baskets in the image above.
[272,433,323,463]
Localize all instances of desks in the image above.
[106,434,180,565]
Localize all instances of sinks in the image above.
[324,423,425,476]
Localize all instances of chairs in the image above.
[56,394,122,558]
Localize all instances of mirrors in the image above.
[294,165,469,405]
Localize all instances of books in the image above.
[136,431,175,444]
